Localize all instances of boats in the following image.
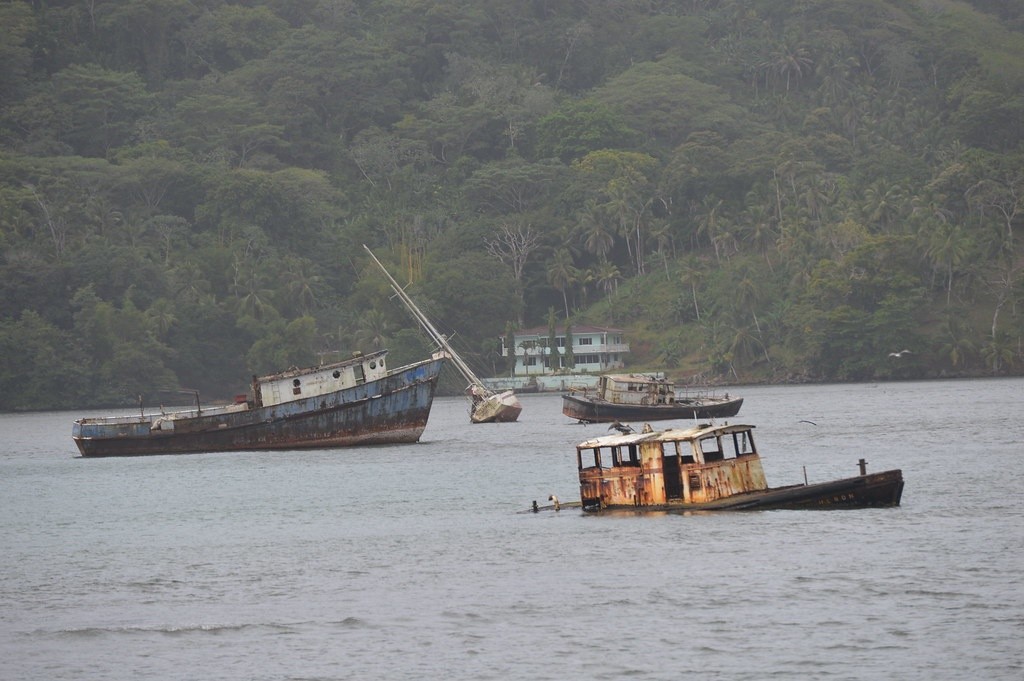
[71,350,442,458]
[515,421,905,513]
[562,370,745,421]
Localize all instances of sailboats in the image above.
[363,244,522,424]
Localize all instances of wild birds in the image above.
[887,349,912,358]
